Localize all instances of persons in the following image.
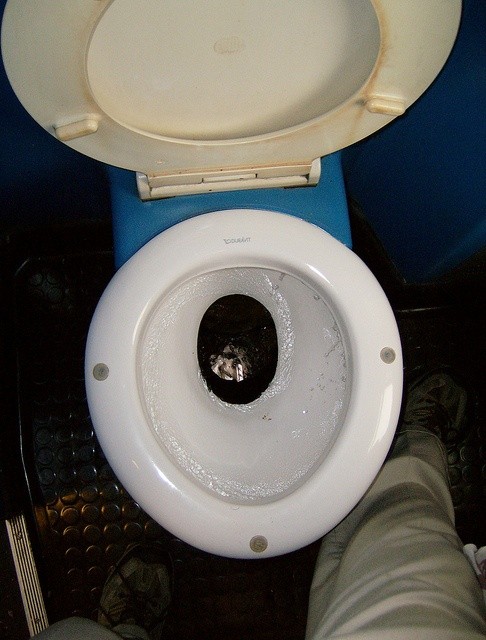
[26,366,486,639]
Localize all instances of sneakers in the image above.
[96,540,172,638]
[399,361,469,446]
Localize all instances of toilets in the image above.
[0,0,461,559]
